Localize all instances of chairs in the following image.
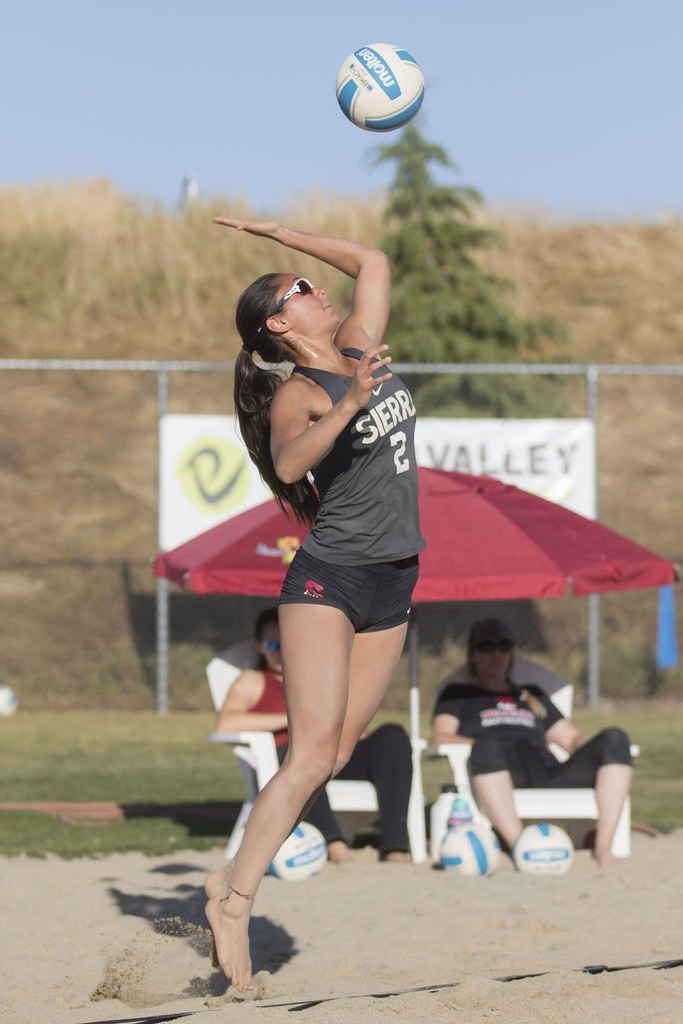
[426,660,641,858]
[205,640,429,860]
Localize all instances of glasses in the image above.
[257,277,314,333]
[475,641,513,653]
[261,639,280,652]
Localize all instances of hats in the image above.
[472,619,515,646]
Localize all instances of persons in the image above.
[432,616,633,869]
[214,607,413,862]
[204,217,427,995]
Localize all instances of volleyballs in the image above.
[268,820,328,880]
[439,823,501,879]
[514,824,575,878]
[333,40,427,132]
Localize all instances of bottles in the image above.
[447,798,473,836]
[430,784,460,862]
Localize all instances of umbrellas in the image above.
[153,467,683,861]
